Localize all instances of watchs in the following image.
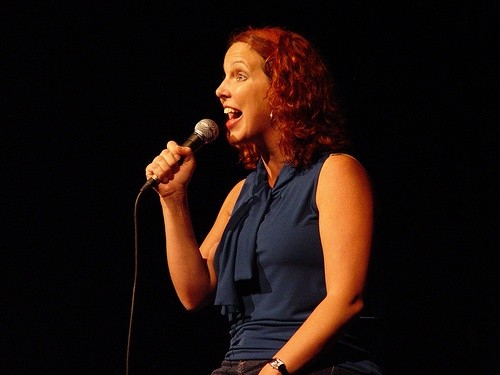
[268,358,290,375]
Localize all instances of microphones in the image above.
[140,119,219,192]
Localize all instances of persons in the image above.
[145,27,383,375]
